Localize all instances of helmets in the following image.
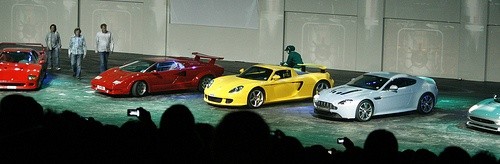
[284,45,295,54]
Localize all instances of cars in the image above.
[313,72,438,122]
[466,94,500,133]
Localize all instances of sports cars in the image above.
[0,43,48,90]
[91,52,225,98]
[204,62,334,109]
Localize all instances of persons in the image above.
[68,28,87,79]
[95,24,114,73]
[45,24,61,70]
[0,52,10,62]
[0,94,500,164]
[280,46,303,68]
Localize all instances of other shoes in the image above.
[77,77,81,81]
[48,66,52,69]
[56,66,61,71]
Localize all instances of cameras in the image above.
[337,138,345,144]
[127,108,141,117]
